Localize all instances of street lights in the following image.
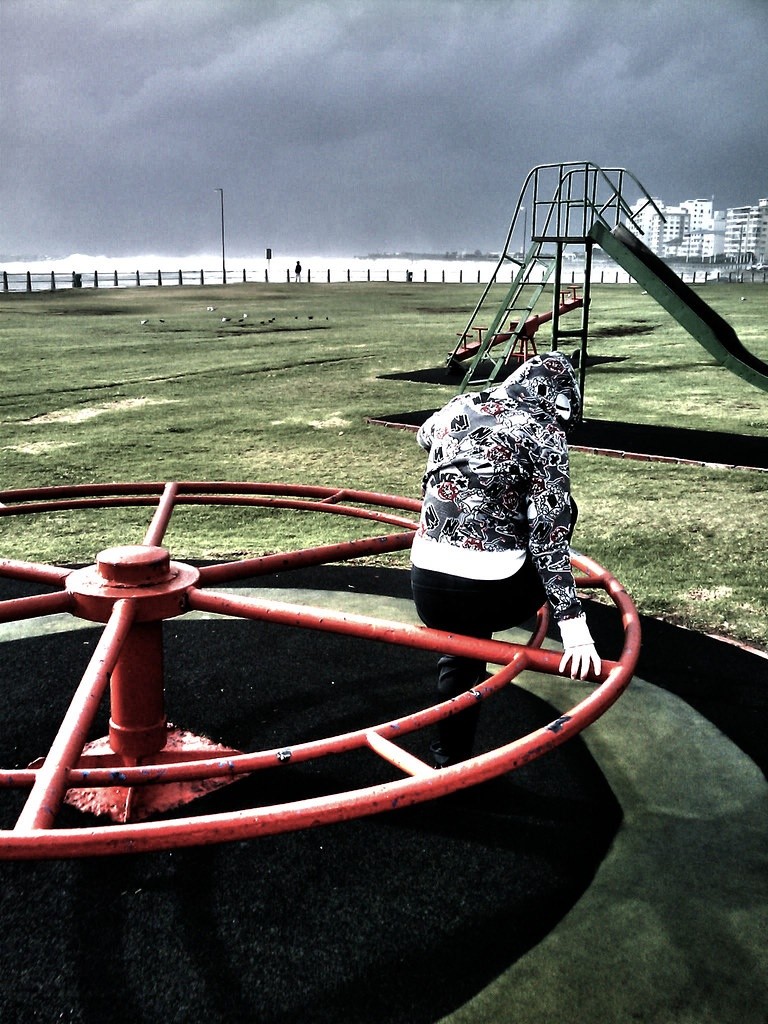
[737,222,743,282]
[684,234,690,264]
[520,207,528,261]
[213,188,225,284]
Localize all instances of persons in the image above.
[411,350,600,770]
[294,261,301,283]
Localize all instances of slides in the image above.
[586,220,768,392]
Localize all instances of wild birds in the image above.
[140,306,329,327]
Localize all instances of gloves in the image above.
[557,612,602,682]
